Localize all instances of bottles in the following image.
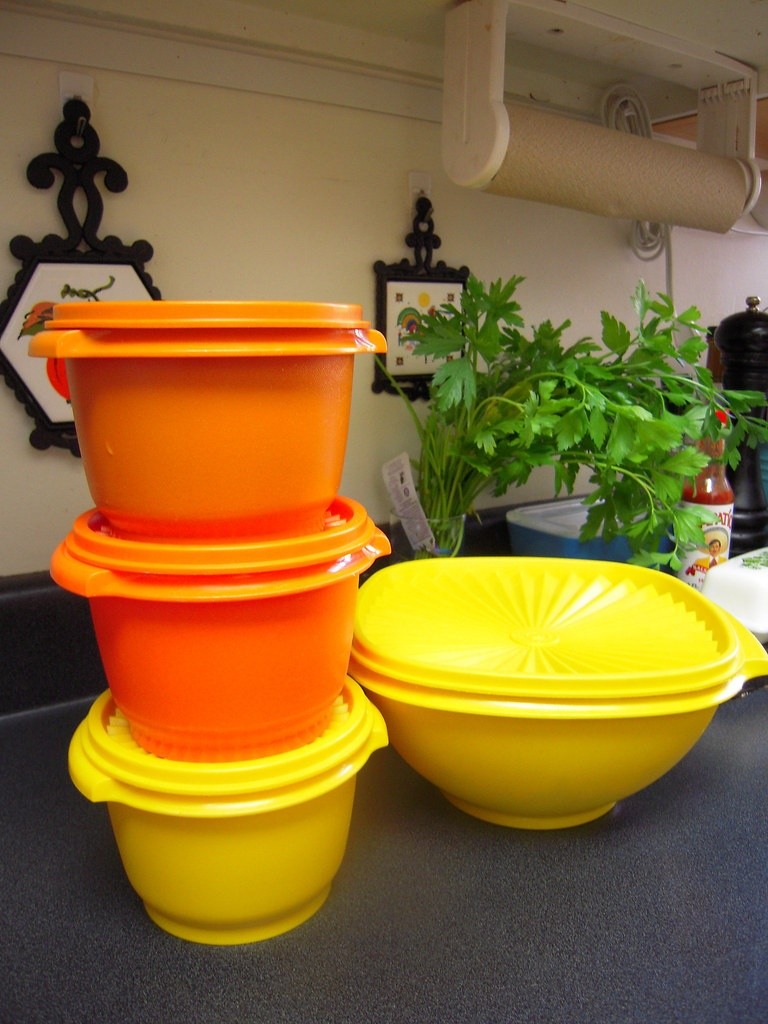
[700,326,728,408]
[671,384,734,592]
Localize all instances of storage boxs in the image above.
[506,497,678,574]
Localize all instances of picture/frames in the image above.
[373,258,471,403]
[0,231,160,458]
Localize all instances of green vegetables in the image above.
[354,272,767,574]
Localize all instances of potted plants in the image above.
[371,273,768,572]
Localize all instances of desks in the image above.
[1,689,768,1024]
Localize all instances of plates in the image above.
[702,546,767,645]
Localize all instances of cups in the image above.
[391,507,465,558]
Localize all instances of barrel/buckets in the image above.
[26,301,391,543]
[66,672,391,943]
[48,495,391,763]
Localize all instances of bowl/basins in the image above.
[349,556,767,831]
